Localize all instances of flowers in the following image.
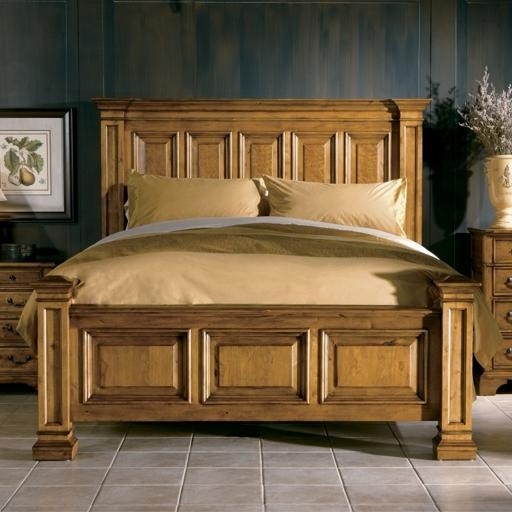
[454,62,512,158]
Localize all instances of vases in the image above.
[482,152,512,232]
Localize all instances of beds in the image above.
[22,94,482,463]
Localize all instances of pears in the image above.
[19,165,35,186]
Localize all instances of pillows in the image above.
[258,171,408,239]
[122,167,267,232]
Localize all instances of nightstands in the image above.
[463,223,512,396]
[0,254,62,396]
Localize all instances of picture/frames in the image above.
[1,102,80,227]
